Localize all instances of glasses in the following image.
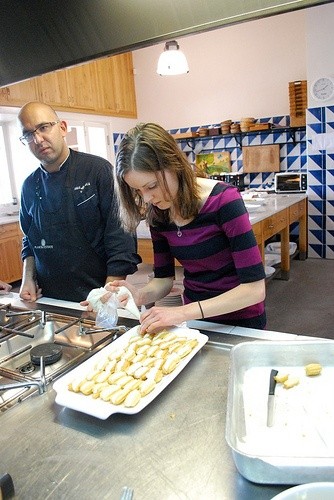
[18,119,59,147]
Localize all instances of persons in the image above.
[103,123,267,330]
[17,101,143,311]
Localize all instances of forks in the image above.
[120,486,134,500]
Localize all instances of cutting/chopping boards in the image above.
[241,145,280,173]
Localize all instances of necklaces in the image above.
[177,226,182,237]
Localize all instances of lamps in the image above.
[156,40,190,77]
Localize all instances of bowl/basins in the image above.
[239,117,255,132]
[198,128,208,137]
[264,266,275,277]
[230,124,240,133]
[221,124,230,134]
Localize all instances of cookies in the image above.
[305,363,322,376]
[273,372,299,388]
[69,328,199,407]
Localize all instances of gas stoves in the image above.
[0,303,127,414]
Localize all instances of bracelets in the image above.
[198,301,204,319]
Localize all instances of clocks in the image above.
[310,76,334,102]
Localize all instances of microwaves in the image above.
[275,172,307,193]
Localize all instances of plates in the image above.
[53,326,209,420]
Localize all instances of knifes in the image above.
[266,369,278,427]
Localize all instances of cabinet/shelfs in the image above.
[135,188,309,280]
[0,52,137,117]
[0,215,27,284]
